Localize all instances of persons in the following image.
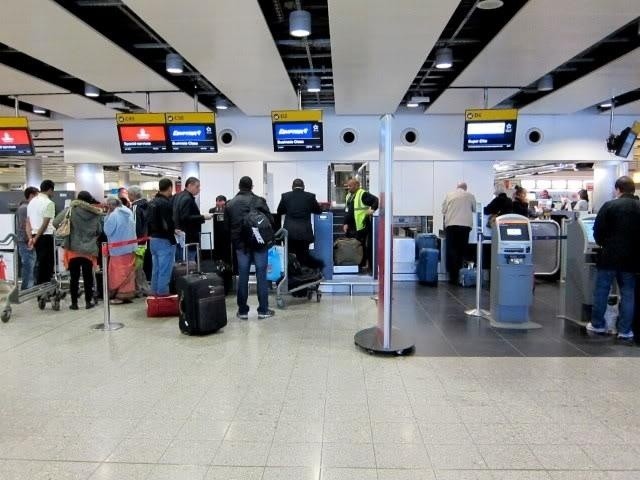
[483,184,512,228]
[514,184,589,219]
[276,178,322,253]
[342,179,378,267]
[585,176,639,338]
[14,175,275,319]
[441,182,476,285]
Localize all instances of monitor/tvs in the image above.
[463,108,518,151]
[165,111,218,154]
[115,112,165,155]
[271,109,324,153]
[0,116,35,157]
[615,127,637,158]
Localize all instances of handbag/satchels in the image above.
[460,265,489,287]
[265,246,285,281]
[54,217,72,238]
[145,294,180,319]
[332,236,365,267]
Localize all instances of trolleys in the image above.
[268,229,324,309]
[54,230,107,304]
[0,234,61,321]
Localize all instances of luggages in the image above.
[168,260,197,295]
[178,275,227,334]
[201,258,231,289]
[416,248,439,287]
[414,231,440,260]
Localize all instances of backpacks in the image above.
[243,205,276,247]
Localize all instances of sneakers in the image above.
[85,302,95,310]
[70,301,80,311]
[257,309,276,319]
[616,327,635,340]
[236,311,249,321]
[585,321,606,333]
[109,298,123,306]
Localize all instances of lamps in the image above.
[215,97,228,110]
[600,99,612,107]
[436,46,453,69]
[33,105,47,114]
[85,81,100,97]
[288,0,311,37]
[166,53,184,73]
[537,75,553,91]
[307,73,321,92]
